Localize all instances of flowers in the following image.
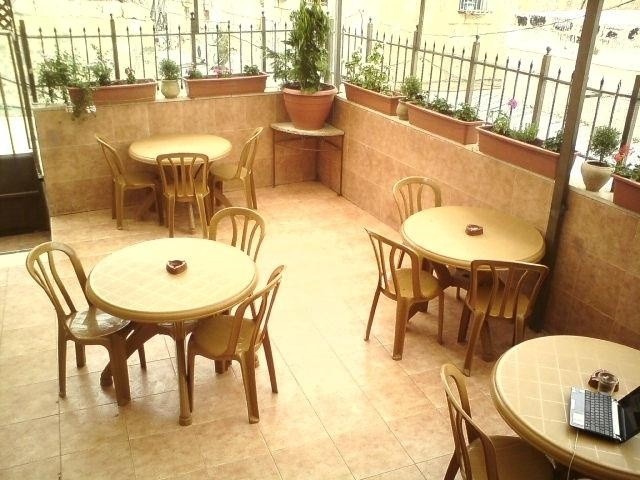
[612,139,640,181]
[476,98,580,143]
[186,61,271,78]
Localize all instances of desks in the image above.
[79,232,260,426]
[488,333,640,479]
[268,115,347,199]
[124,132,232,224]
[400,209,548,346]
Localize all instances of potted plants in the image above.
[26,42,158,124]
[341,42,399,114]
[391,71,422,121]
[404,96,486,149]
[156,56,183,100]
[274,0,340,129]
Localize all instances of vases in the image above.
[607,173,640,214]
[578,124,619,196]
[187,73,267,101]
[473,127,561,181]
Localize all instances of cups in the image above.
[597,372,619,397]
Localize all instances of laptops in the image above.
[569,385,640,443]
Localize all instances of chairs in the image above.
[210,126,267,211]
[94,128,164,231]
[437,358,556,480]
[356,220,452,363]
[187,200,268,380]
[187,261,289,425]
[452,249,547,379]
[26,240,151,416]
[156,152,218,241]
[392,178,461,300]
